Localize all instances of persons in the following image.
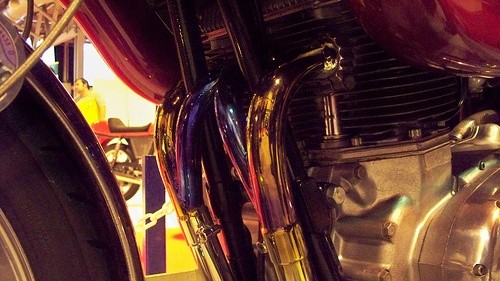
[72,77,106,130]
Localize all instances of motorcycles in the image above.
[0,0,498,281]
